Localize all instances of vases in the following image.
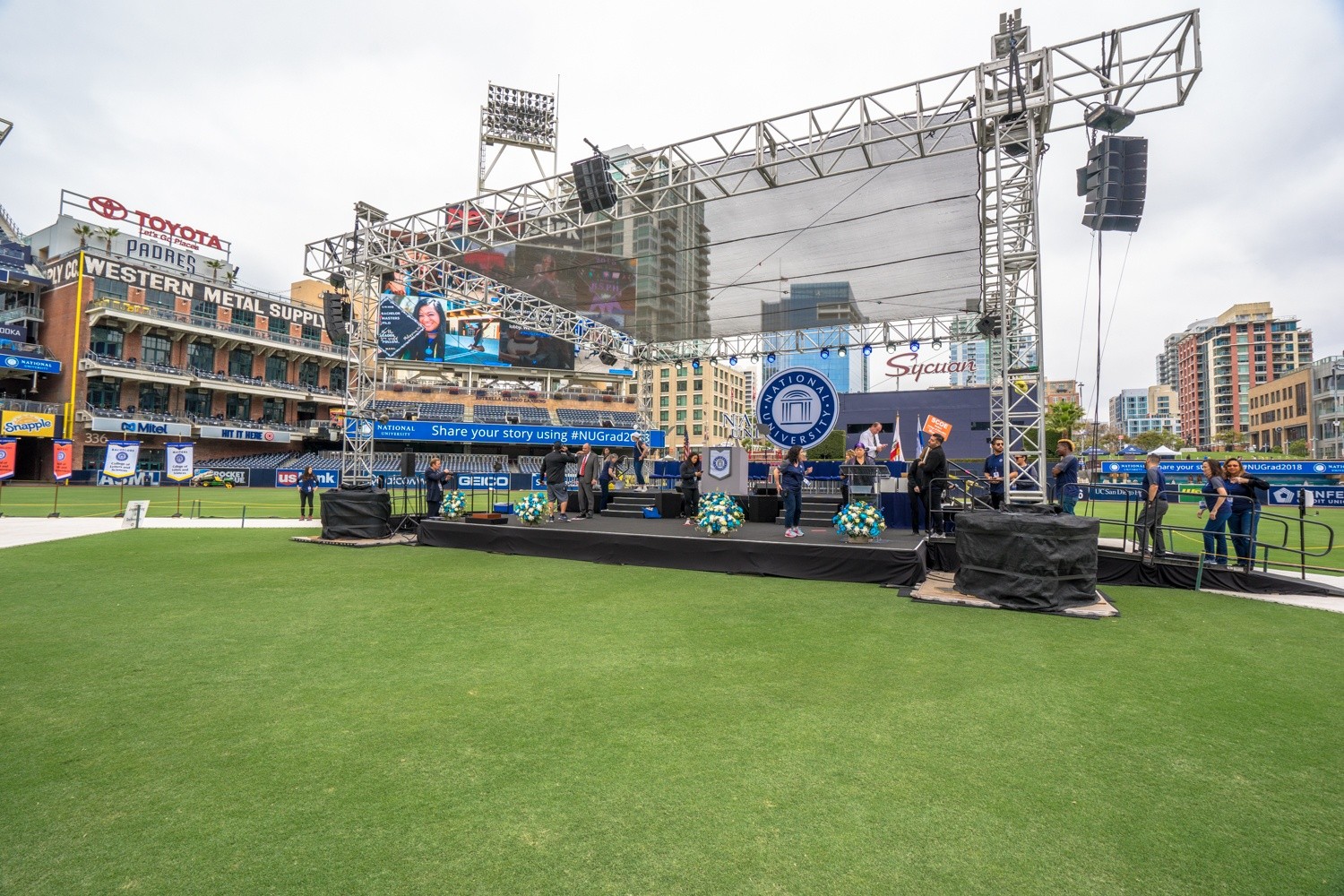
[848,535,869,543]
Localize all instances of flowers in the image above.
[831,500,893,538]
[436,489,469,520]
[695,488,748,535]
[512,494,562,526]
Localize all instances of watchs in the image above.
[1148,500,1153,504]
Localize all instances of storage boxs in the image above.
[495,502,514,513]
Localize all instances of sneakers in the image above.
[558,514,571,522]
[1203,558,1218,565]
[642,486,648,492]
[784,528,797,538]
[545,515,555,523]
[794,526,805,536]
[633,486,642,491]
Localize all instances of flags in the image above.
[890,417,905,462]
[683,428,691,460]
[915,418,924,459]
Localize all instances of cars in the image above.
[192,472,236,488]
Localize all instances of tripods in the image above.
[389,477,420,539]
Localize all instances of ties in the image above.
[873,435,878,455]
[581,456,586,475]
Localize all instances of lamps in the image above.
[931,338,942,350]
[820,348,830,359]
[886,342,896,353]
[751,353,758,363]
[910,340,920,352]
[598,352,618,365]
[675,360,682,369]
[692,359,699,369]
[767,352,775,363]
[837,346,846,357]
[710,357,717,367]
[862,344,872,356]
[976,315,996,337]
[729,355,737,365]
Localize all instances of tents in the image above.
[1147,445,1182,460]
[1114,445,1147,460]
[1082,446,1111,459]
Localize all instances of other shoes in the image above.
[685,518,690,525]
[307,516,313,521]
[299,515,304,521]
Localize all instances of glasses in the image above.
[1016,457,1023,459]
[997,443,1004,446]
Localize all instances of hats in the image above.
[632,433,640,439]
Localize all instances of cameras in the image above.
[447,472,453,476]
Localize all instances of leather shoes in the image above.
[586,513,593,518]
[576,512,586,518]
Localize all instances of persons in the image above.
[984,437,1079,515]
[908,433,958,538]
[839,421,888,506]
[1196,457,1271,571]
[425,458,453,518]
[1135,454,1169,558]
[541,432,648,523]
[773,445,812,538]
[380,245,446,297]
[403,298,446,362]
[296,466,319,521]
[681,452,703,526]
[532,255,560,302]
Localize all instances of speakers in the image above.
[401,453,415,477]
[655,493,682,518]
[749,496,779,523]
[573,158,619,214]
[323,292,347,344]
[1083,136,1148,233]
